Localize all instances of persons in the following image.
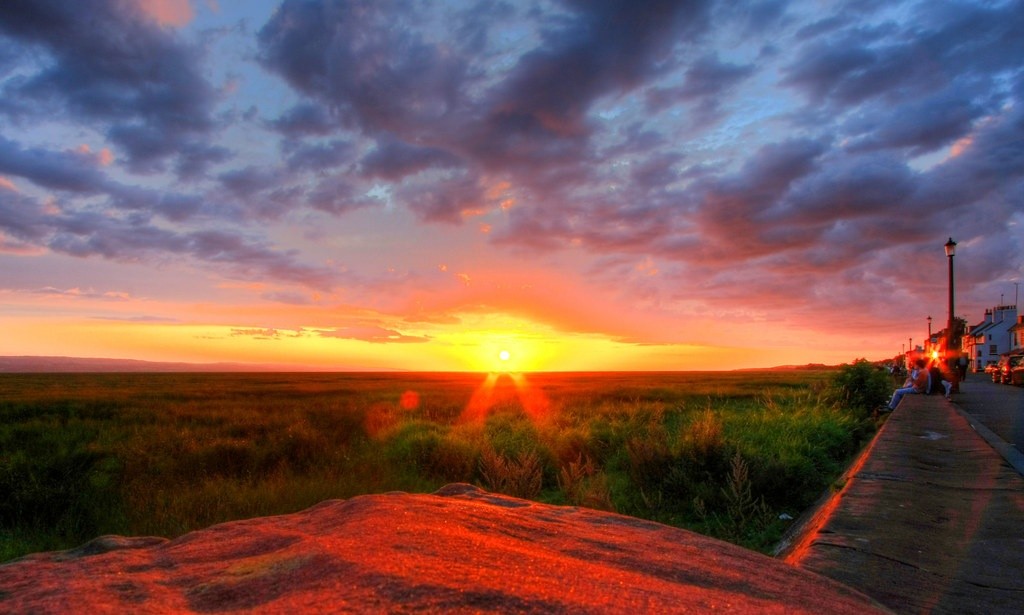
[877,360,952,412]
[956,351,970,383]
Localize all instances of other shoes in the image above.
[877,407,892,412]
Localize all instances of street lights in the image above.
[944,237,958,337]
[927,316,933,355]
[1001,294,1004,306]
[908,337,912,351]
[902,344,905,367]
[1014,282,1020,305]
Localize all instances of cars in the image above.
[986,349,1024,386]
